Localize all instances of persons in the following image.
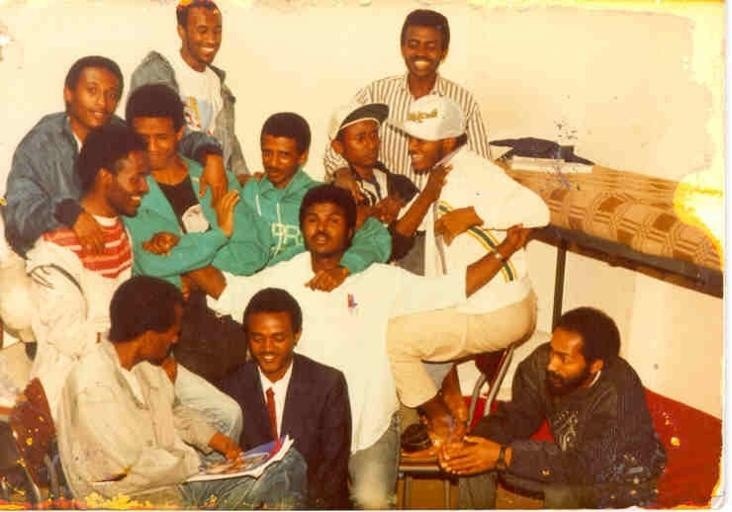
[437,304,665,509]
[53,275,309,509]
[319,8,492,193]
[2,56,234,361]
[121,84,452,380]
[383,94,553,452]
[217,287,354,509]
[28,125,244,461]
[140,181,532,509]
[121,1,248,187]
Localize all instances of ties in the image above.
[266,388,278,445]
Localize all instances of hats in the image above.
[327,98,391,141]
[392,94,466,142]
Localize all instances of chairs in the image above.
[6,376,62,510]
[396,340,516,508]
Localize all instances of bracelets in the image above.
[494,442,509,472]
[490,248,507,262]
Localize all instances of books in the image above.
[185,434,294,480]
